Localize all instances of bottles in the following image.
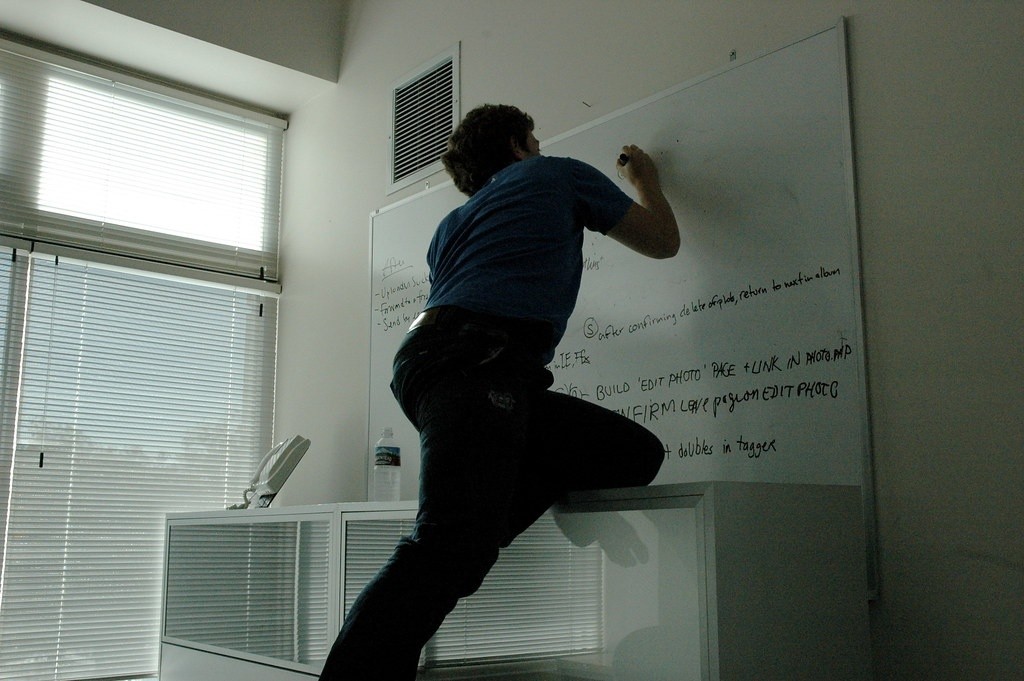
[372,427,402,501]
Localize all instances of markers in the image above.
[619,153,627,165]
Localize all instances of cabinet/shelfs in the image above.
[153,481,874,680]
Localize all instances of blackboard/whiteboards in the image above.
[366,12,880,602]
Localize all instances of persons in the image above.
[317,105,681,681]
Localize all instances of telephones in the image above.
[250,434,312,496]
